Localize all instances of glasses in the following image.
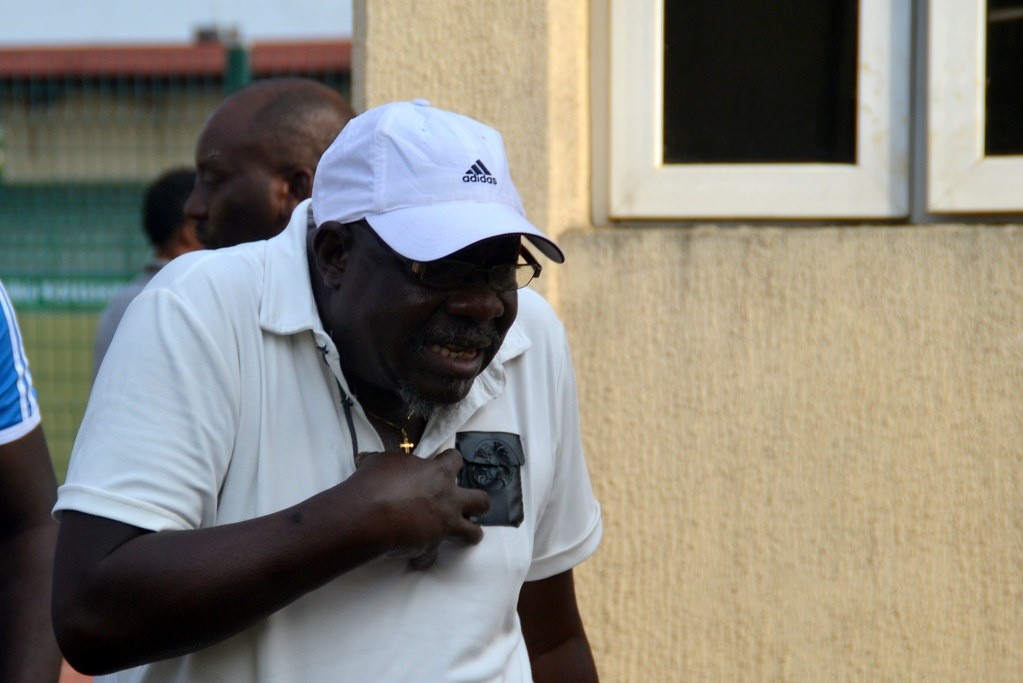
[356,220,542,293]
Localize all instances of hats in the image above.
[311,100,566,263]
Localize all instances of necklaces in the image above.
[366,407,419,455]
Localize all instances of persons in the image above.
[93,169,196,379]
[50,100,603,683]
[180,78,360,248]
[0,284,63,683]
